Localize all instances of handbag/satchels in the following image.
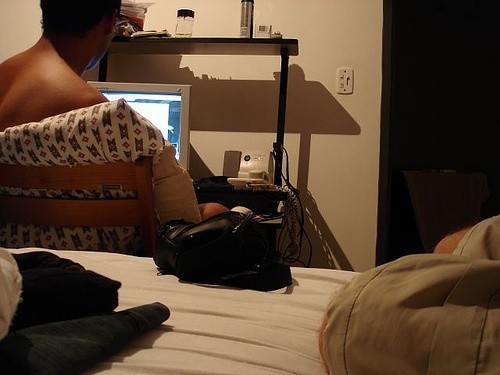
[153,211,292,291]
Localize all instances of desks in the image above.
[238,189,290,260]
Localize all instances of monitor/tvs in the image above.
[88,81,192,172]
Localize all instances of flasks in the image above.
[240,0,255,39]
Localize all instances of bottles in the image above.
[175,9,194,38]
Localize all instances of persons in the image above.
[318,214,500,375]
[0,0,230,221]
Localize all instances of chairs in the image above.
[0,160,159,245]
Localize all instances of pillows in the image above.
[0,100,202,248]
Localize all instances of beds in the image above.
[0,251,363,375]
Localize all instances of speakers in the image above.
[222,150,242,177]
